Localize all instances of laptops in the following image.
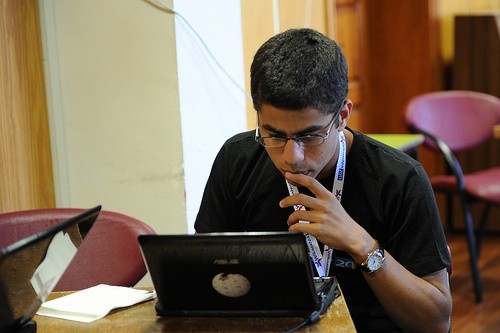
[0,205,102,333]
[137,230,338,318]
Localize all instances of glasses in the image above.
[255,100,345,148]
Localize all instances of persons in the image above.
[193,28,454,333]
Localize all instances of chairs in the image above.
[0,209,155,291]
[404,91,500,304]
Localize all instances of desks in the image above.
[365,133,423,160]
[32,276,359,333]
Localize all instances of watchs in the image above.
[351,238,386,274]
